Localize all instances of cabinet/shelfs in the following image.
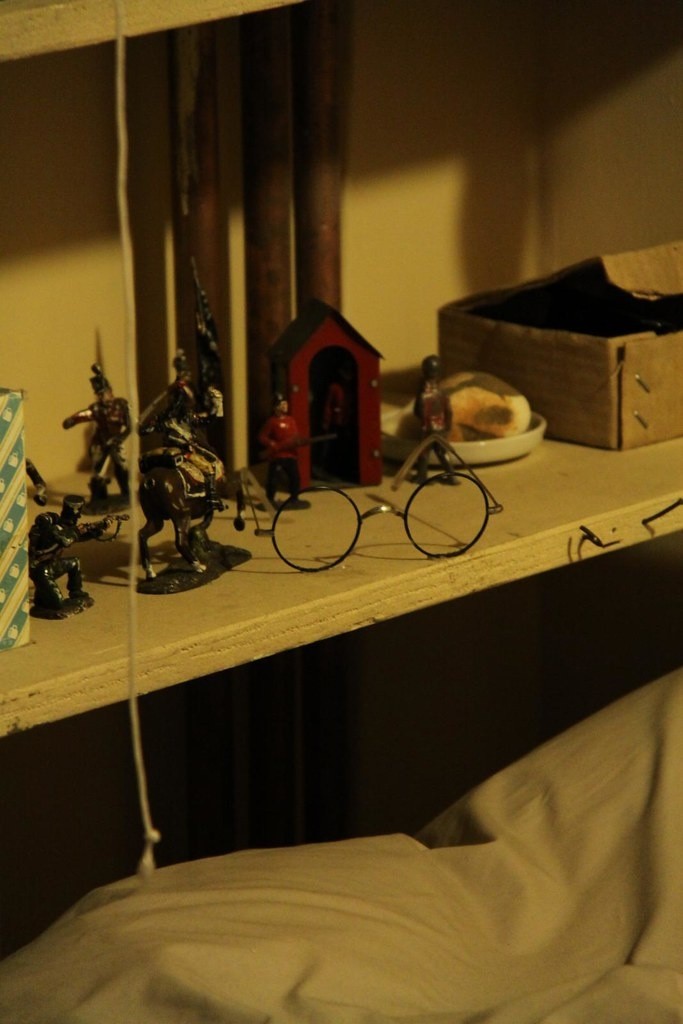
[0,0,683,739]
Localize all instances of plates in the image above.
[379,407,548,468]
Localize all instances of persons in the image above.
[29,495,114,610]
[64,375,132,504]
[155,352,229,511]
[259,394,302,504]
[313,367,354,483]
[410,356,459,485]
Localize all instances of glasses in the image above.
[239,434,505,573]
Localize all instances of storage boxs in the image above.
[1,386,32,651]
[437,239,683,452]
